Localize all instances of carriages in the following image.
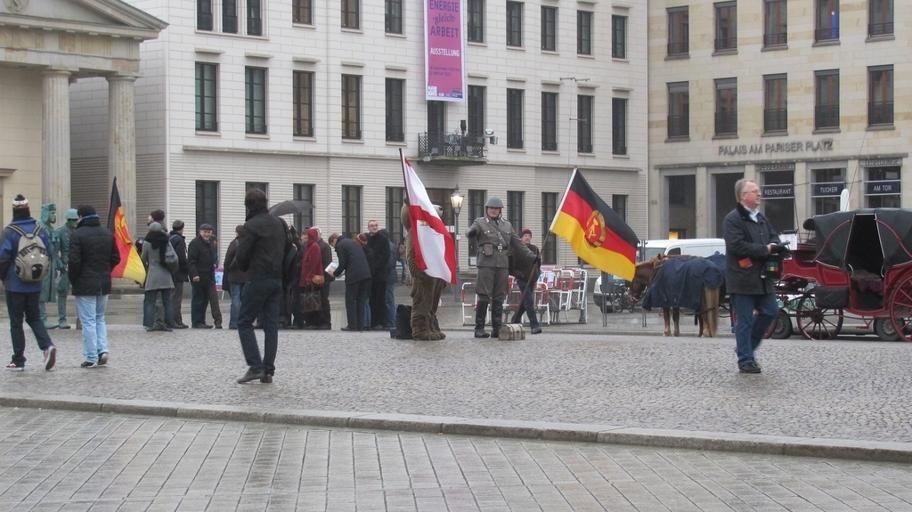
[626,207,911,342]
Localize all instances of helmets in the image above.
[484,196,505,209]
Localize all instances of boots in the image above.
[511,304,526,324]
[491,300,504,338]
[474,299,490,339]
[525,304,542,335]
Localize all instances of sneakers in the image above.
[4,360,25,371]
[43,345,57,372]
[80,360,98,369]
[260,373,274,385]
[98,352,109,366]
[735,347,762,374]
[339,323,395,331]
[237,366,266,384]
[143,321,331,332]
[415,331,446,341]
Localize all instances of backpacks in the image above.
[5,222,51,283]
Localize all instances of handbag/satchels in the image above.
[299,281,323,314]
[163,239,180,274]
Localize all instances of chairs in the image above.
[440,133,485,157]
[459,261,592,329]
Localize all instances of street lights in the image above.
[448,179,464,300]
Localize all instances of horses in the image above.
[626,253,721,338]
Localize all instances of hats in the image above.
[198,222,215,230]
[172,219,185,231]
[12,193,30,215]
[148,222,163,233]
[356,232,369,247]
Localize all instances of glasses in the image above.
[740,188,763,195]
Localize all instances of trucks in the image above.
[591,232,726,316]
